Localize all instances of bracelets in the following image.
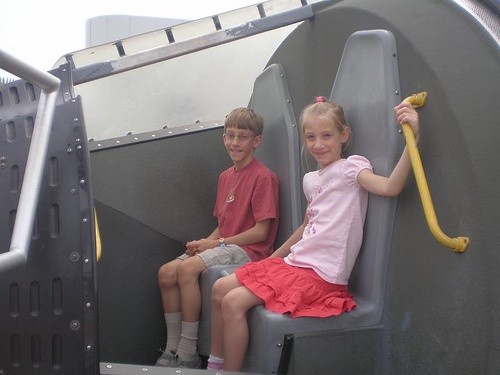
[219,237,225,245]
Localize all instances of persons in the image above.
[156,96,419,373]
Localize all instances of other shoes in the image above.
[155,350,201,369]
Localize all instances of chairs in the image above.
[198,28,402,375]
